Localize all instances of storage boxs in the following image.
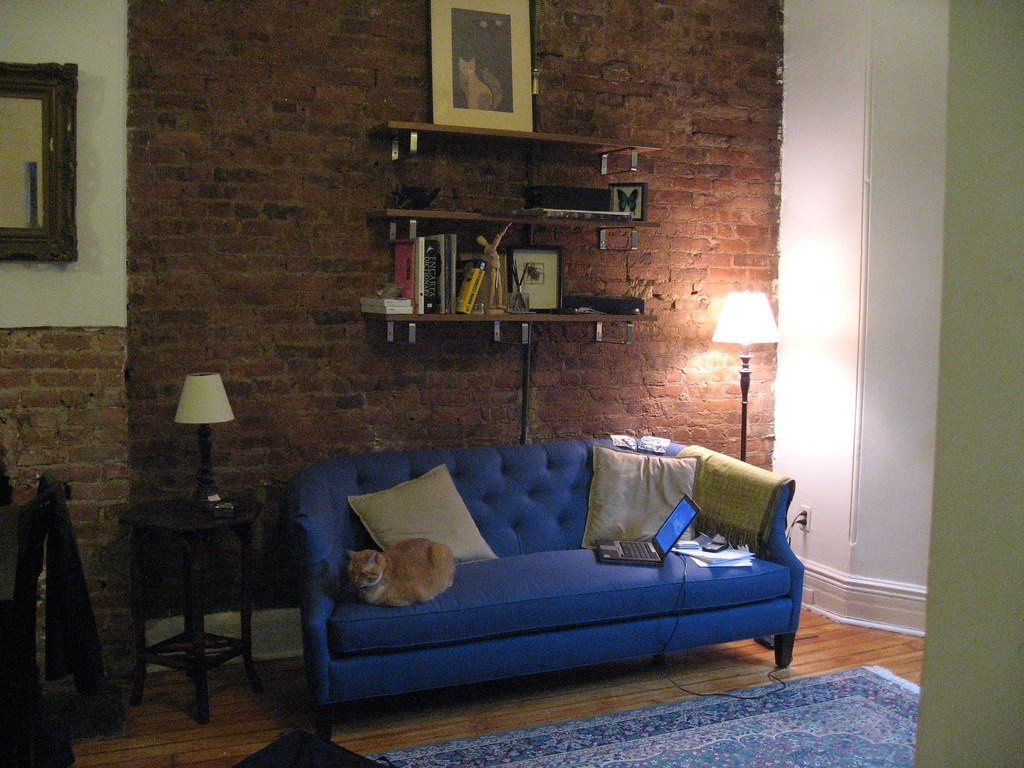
[361,306,413,314]
[360,297,411,307]
[524,184,612,212]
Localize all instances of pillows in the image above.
[349,463,498,567]
[582,442,700,554]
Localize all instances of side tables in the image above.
[119,502,265,723]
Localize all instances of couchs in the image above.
[282,439,805,744]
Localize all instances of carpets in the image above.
[366,662,921,768]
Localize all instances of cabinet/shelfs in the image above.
[359,120,662,345]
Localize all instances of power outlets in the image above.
[798,504,810,533]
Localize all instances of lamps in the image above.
[175,373,235,518]
[713,289,781,462]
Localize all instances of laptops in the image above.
[595,494,702,566]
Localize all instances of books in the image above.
[360,232,485,316]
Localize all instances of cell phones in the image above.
[701,541,729,552]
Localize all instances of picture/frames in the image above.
[427,0,538,133]
[0,60,79,265]
[508,244,566,313]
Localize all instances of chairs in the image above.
[0,470,56,768]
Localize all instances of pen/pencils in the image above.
[511,260,529,313]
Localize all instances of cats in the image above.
[343,538,457,607]
